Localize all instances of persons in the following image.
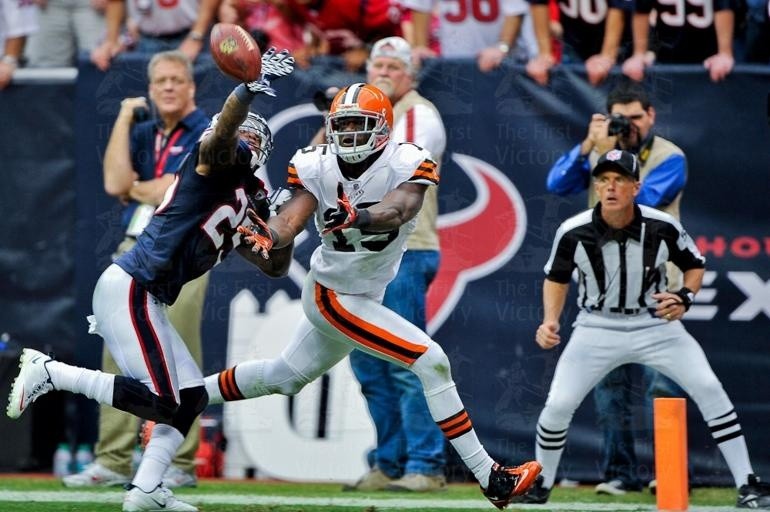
[545,82,692,497]
[0,44,298,511]
[140,79,542,512]
[59,51,212,491]
[508,150,770,512]
[311,38,450,493]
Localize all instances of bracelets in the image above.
[665,286,695,313]
[122,180,140,204]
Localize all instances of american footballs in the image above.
[210,22,261,82]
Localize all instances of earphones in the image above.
[188,88,195,99]
[149,91,154,100]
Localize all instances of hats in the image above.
[370,37,411,66]
[591,149,638,181]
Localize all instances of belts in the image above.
[587,306,641,314]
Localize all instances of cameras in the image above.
[607,115,632,136]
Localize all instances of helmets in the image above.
[325,83,394,164]
[198,110,271,171]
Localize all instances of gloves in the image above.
[269,187,291,214]
[319,183,368,235]
[233,46,295,105]
[237,209,277,260]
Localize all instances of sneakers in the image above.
[480,460,542,510]
[736,476,770,509]
[649,479,657,494]
[123,482,199,512]
[62,463,198,486]
[507,475,550,503]
[7,349,55,419]
[385,474,447,493]
[342,464,390,492]
[594,480,642,495]
